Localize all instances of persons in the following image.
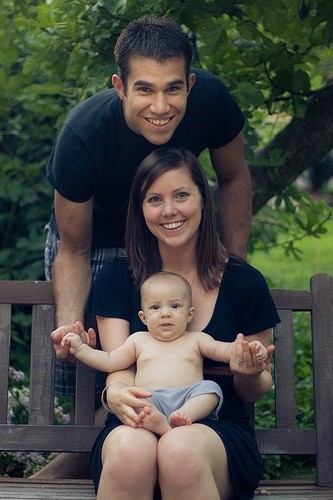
[59,269,270,436]
[26,14,253,485]
[91,147,285,500]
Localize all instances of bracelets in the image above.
[100,380,129,414]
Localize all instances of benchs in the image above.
[0,273,333,500]
